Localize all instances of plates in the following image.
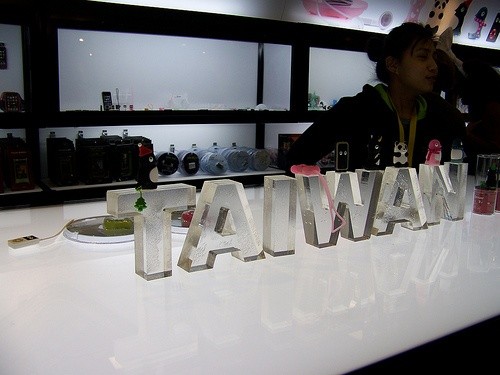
[65,214,135,244]
[303,0,369,19]
[171,218,189,233]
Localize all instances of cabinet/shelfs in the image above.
[0,0,500,207]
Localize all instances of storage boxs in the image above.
[0,130,155,194]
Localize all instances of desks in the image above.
[0,177,500,375]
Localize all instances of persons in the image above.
[276,22,476,178]
[433,49,500,168]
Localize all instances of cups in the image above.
[472,155,500,216]
